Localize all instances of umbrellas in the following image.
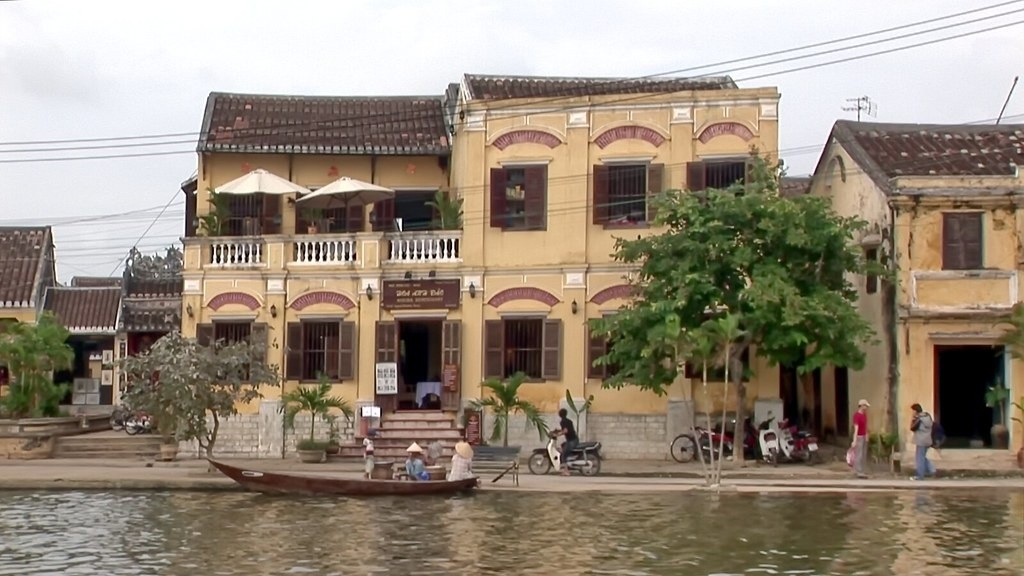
[214,168,310,233]
[293,176,396,231]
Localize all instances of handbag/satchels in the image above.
[926,412,946,449]
[846,447,855,467]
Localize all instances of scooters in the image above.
[527,428,607,477]
[670,416,821,467]
[108,402,161,435]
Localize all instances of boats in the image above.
[204,456,483,496]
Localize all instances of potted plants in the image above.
[324,415,339,454]
[269,367,356,465]
[300,204,325,233]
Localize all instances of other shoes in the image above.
[910,476,918,481]
[561,471,572,476]
[925,472,936,477]
[857,473,872,479]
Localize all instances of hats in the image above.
[859,399,870,406]
[455,441,474,458]
[406,442,423,452]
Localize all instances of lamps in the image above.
[449,124,457,137]
[460,110,465,122]
[187,302,194,317]
[270,304,277,318]
[469,281,475,298]
[572,298,577,314]
[366,284,372,300]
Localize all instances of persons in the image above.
[403,443,427,481]
[909,403,937,481]
[850,399,870,478]
[549,408,580,475]
[445,441,475,482]
